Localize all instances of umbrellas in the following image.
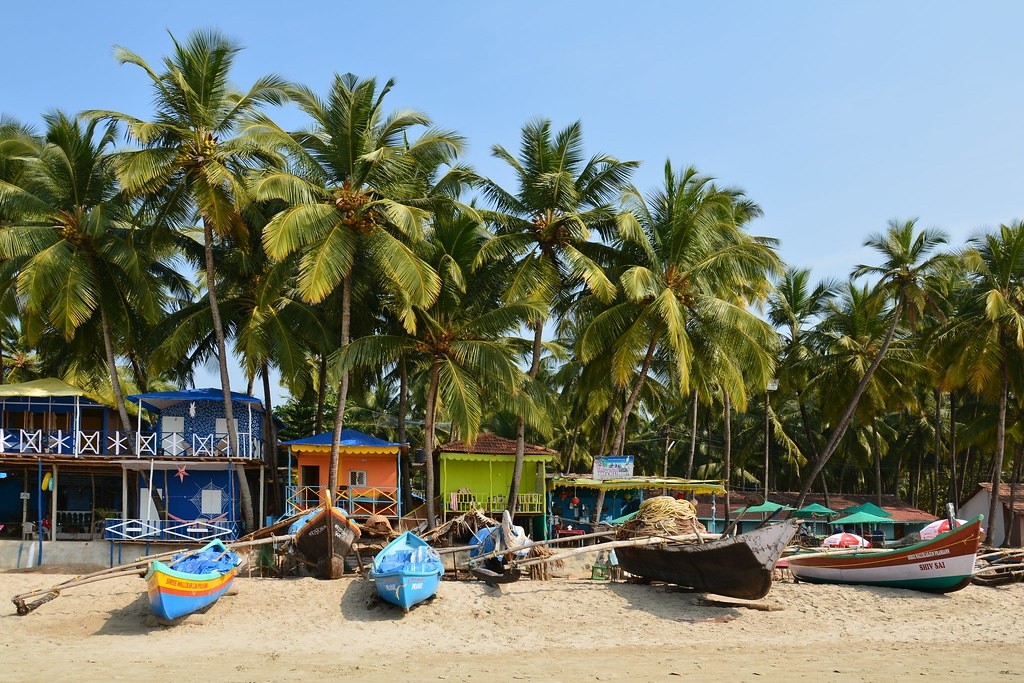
[843,502,893,518]
[728,500,797,528]
[827,511,896,550]
[791,503,839,536]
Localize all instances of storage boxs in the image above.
[592,566,607,580]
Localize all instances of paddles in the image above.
[9,531,303,621]
[775,547,897,566]
[971,546,1024,578]
[457,527,620,570]
[500,531,732,574]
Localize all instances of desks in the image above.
[812,534,828,547]
[451,492,475,510]
[351,496,379,514]
[553,530,587,547]
[518,494,542,511]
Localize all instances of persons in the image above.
[806,523,811,533]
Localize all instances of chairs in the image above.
[377,492,395,514]
[336,491,348,511]
[21,522,36,540]
[43,528,52,541]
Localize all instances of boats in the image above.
[144,538,242,622]
[287,489,361,579]
[776,512,985,594]
[370,530,445,613]
[466,509,531,584]
[609,514,801,600]
